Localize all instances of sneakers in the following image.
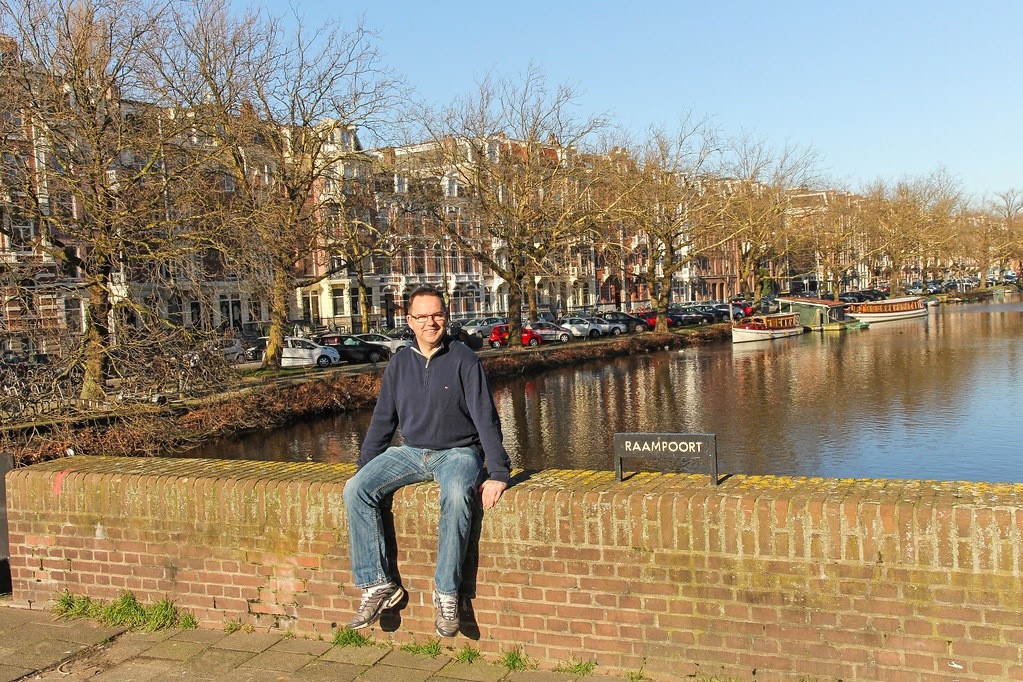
[432,590,459,638]
[350,580,404,629]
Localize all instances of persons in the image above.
[381,329,384,334]
[535,318,544,328]
[342,288,512,637]
[443,289,823,350]
[526,318,531,326]
[398,330,415,339]
[369,326,375,333]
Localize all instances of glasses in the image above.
[410,310,446,323]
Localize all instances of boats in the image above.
[844,298,929,323]
[731,313,803,343]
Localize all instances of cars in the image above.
[247,336,339,367]
[319,335,391,362]
[595,311,646,332]
[521,322,573,343]
[694,306,730,322]
[559,317,602,339]
[183,339,246,365]
[713,304,745,318]
[732,302,756,316]
[836,271,1016,303]
[489,324,543,348]
[671,307,715,326]
[583,316,628,337]
[461,317,503,338]
[628,312,673,327]
[354,334,412,354]
